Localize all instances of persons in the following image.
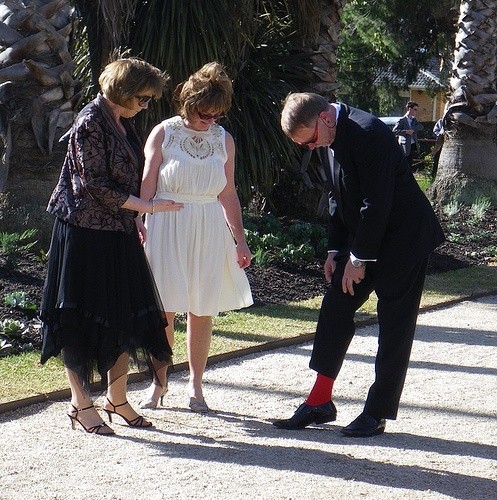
[392,101,424,166]
[38,58,183,438]
[430,118,444,177]
[272,92,446,437]
[137,62,253,412]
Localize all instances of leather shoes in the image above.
[340,412,387,437]
[272,399,337,430]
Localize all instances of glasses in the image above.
[297,116,319,147]
[412,108,418,111]
[133,95,152,108]
[196,107,224,120]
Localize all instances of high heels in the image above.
[102,397,152,428]
[185,384,209,413]
[67,402,115,435]
[140,382,169,409]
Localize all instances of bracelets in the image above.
[149,198,154,215]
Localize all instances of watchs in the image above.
[350,256,364,267]
